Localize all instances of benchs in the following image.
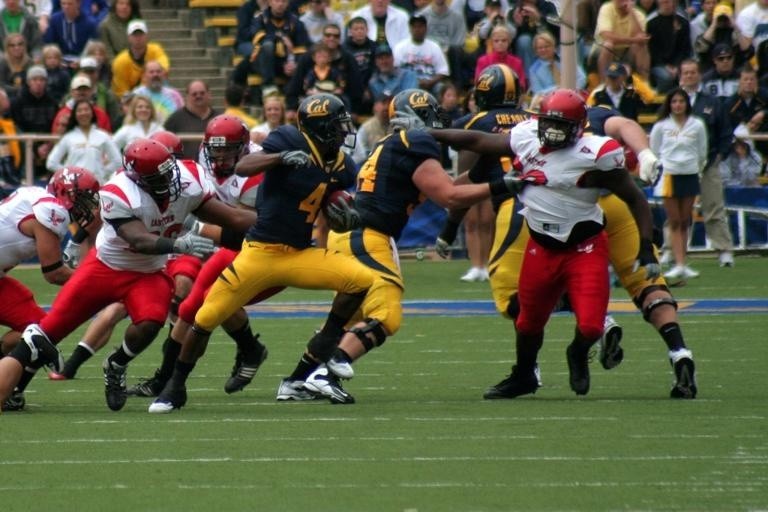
[188,1,316,107]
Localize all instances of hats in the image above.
[26,66,47,82]
[127,17,148,36]
[713,5,732,17]
[374,45,392,57]
[485,0,501,7]
[604,62,627,77]
[80,57,97,68]
[712,43,733,59]
[408,14,427,24]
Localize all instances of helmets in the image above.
[48,167,100,227]
[536,89,589,145]
[389,89,443,129]
[525,87,554,115]
[122,140,182,205]
[202,113,250,176]
[298,94,357,162]
[474,65,522,111]
[149,130,185,158]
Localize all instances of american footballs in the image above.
[326,190,354,211]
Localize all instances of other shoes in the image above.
[658,253,676,264]
[683,266,699,279]
[719,253,734,267]
[661,266,683,278]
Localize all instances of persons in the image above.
[49,130,225,384]
[543,95,701,397]
[590,1,768,187]
[39,98,123,264]
[1,137,259,412]
[663,61,735,266]
[109,79,221,156]
[148,94,373,414]
[1,162,104,374]
[1,1,216,175]
[387,83,661,398]
[437,185,471,260]
[458,0,590,107]
[455,80,503,283]
[438,64,625,396]
[124,112,262,416]
[277,88,526,403]
[241,1,458,112]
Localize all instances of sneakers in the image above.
[102,348,128,411]
[0,392,24,412]
[126,368,164,396]
[325,355,355,382]
[462,267,491,283]
[535,368,542,386]
[483,365,538,398]
[21,326,64,374]
[277,377,319,401]
[598,315,623,370]
[147,385,188,413]
[666,349,698,400]
[224,334,268,394]
[48,362,76,380]
[302,363,356,405]
[564,341,597,396]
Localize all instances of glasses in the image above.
[323,32,340,39]
[494,39,506,42]
[717,55,732,61]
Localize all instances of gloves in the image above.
[631,252,661,282]
[636,148,658,182]
[389,104,431,134]
[279,149,317,170]
[327,196,361,227]
[490,167,528,195]
[172,220,214,259]
